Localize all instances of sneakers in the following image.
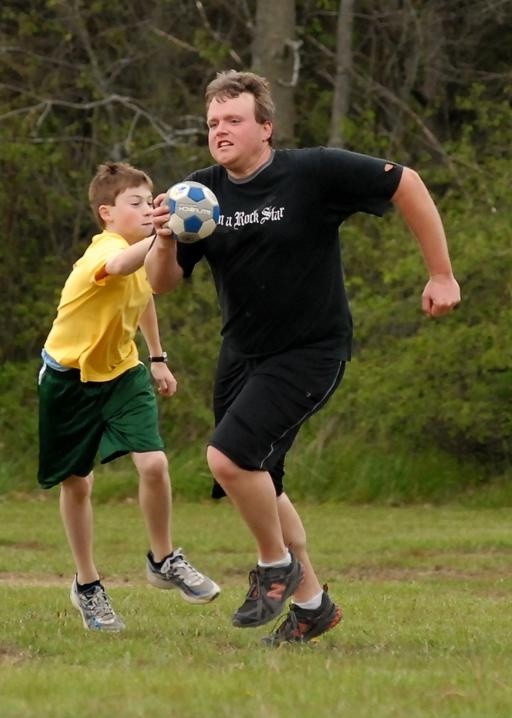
[233,551,306,628]
[144,549,220,604]
[70,573,123,634]
[261,585,344,650]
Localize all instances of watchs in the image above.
[149,351,168,363]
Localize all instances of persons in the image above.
[35,159,223,635]
[144,70,461,647]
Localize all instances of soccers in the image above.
[160,180,220,243]
